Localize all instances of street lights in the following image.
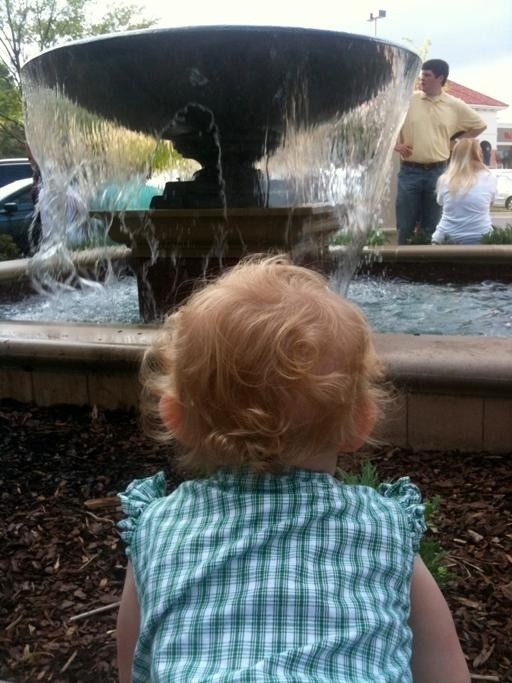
[366,10,386,36]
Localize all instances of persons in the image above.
[116,254,473,682]
[430,136,498,244]
[391,56,487,246]
[25,142,90,254]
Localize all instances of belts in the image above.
[399,158,447,169]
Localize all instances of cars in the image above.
[0,158,42,251]
[489,168,512,209]
